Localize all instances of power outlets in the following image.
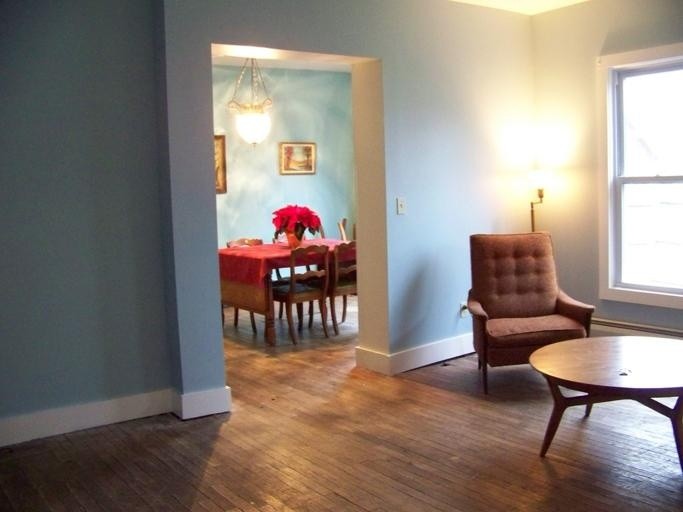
[460,303,470,319]
[465,230,595,396]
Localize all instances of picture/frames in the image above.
[214,134,227,196]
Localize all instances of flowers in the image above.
[268,204,323,243]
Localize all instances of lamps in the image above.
[527,179,546,233]
[278,140,318,176]
[226,56,274,146]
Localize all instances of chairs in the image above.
[228,238,357,345]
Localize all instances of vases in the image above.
[285,228,304,249]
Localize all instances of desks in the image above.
[528,333,682,474]
[218,235,356,344]
